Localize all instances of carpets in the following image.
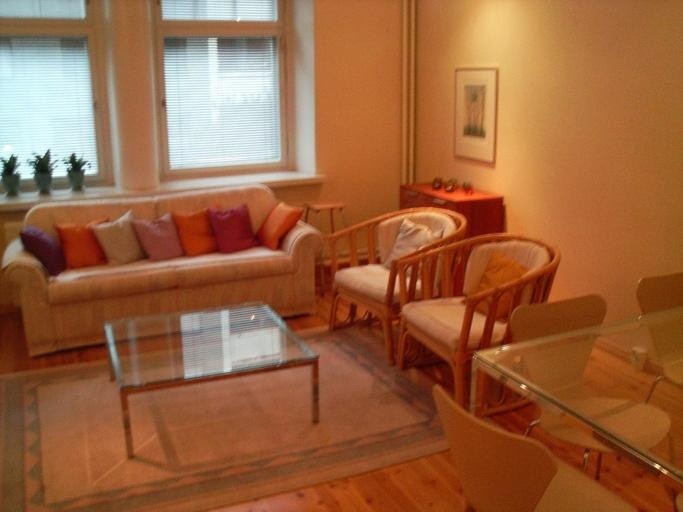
[0,327,452,512]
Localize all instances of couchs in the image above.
[0,183,322,358]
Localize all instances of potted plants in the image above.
[63,152,89,192]
[0,152,22,197]
[27,148,58,194]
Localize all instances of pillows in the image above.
[384,217,444,279]
[462,251,526,323]
[19,202,303,275]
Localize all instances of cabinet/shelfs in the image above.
[400,179,504,293]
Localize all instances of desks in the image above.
[468,304,683,481]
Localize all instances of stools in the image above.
[304,199,353,298]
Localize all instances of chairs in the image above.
[635,272,683,399]
[395,232,561,416]
[323,207,468,364]
[509,294,677,512]
[432,381,637,512]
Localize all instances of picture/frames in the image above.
[453,67,498,165]
